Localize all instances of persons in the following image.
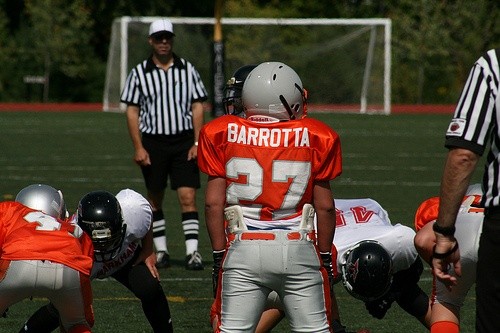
[256,198,432,333]
[18,189,173,333]
[119,20,209,269]
[0,184,95,333]
[197,62,340,333]
[414,183,484,333]
[432,46,500,333]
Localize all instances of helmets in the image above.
[231,65,259,115]
[76,191,123,253]
[342,244,392,300]
[16,184,68,221]
[244,62,304,121]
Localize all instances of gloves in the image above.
[209,249,226,298]
[318,251,337,294]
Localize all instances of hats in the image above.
[148,19,176,37]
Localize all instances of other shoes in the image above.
[184,251,203,270]
[154,250,172,268]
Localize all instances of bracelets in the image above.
[433,220,455,236]
[194,142,198,146]
